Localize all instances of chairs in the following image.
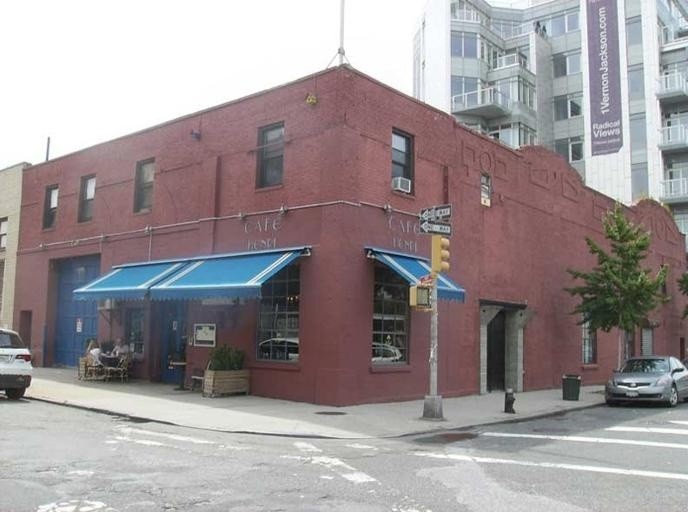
[78,351,133,384]
[191,360,213,391]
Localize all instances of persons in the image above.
[87,338,130,382]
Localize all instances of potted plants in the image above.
[202,343,250,399]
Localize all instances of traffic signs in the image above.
[418,220,453,236]
[419,204,453,219]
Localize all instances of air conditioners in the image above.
[391,177,411,193]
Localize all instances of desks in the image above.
[169,361,193,391]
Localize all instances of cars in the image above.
[0,328,33,403]
[260,337,300,360]
[371,341,403,365]
[603,355,687,405]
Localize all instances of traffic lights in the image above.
[430,234,450,271]
[409,285,431,308]
